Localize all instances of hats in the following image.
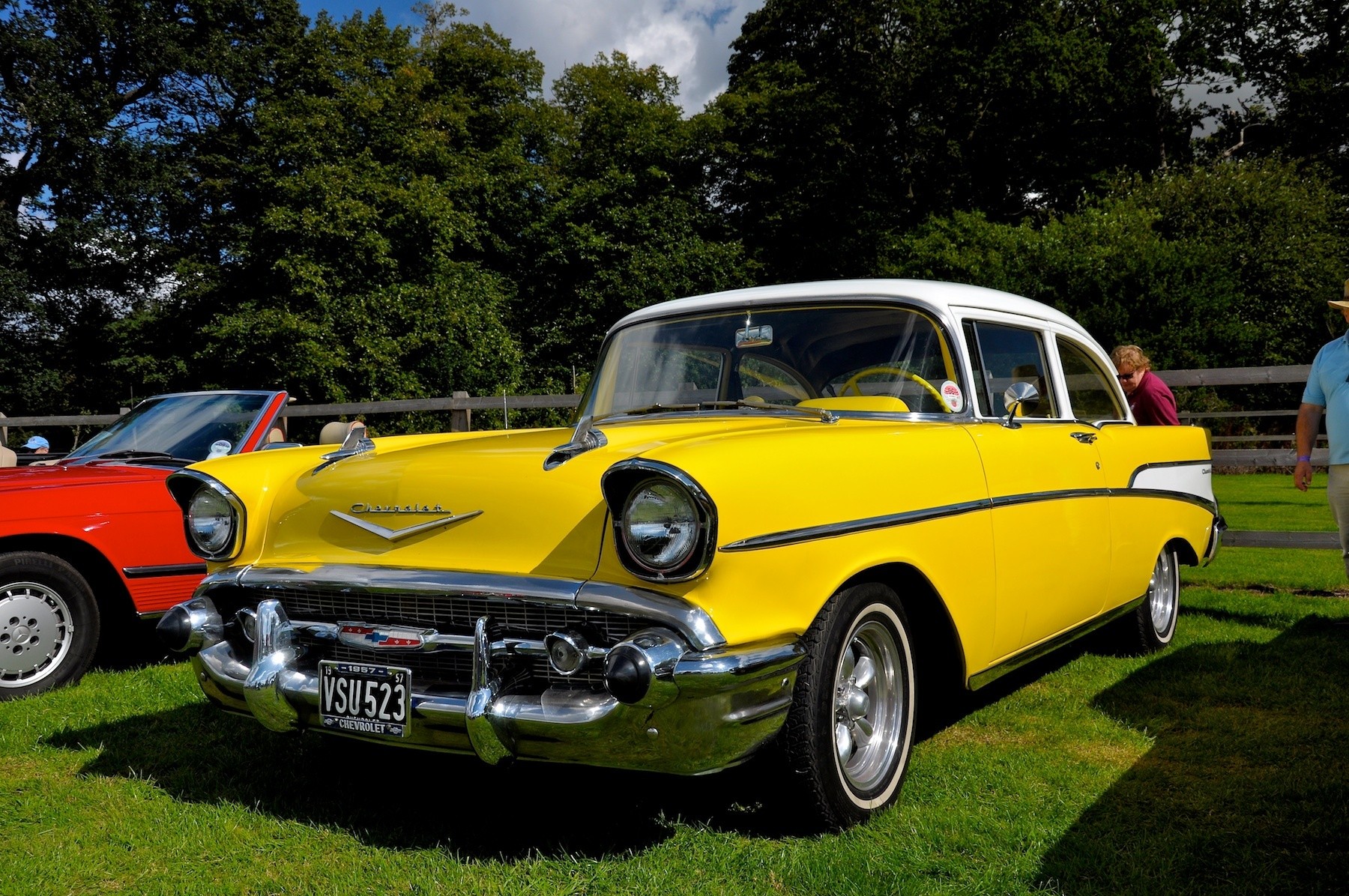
[1327,278,1349,309]
[20,436,50,450]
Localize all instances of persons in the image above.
[1294,279,1349,578]
[1110,345,1180,425]
[19,436,50,455]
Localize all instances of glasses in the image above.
[1116,369,1135,380]
[26,448,36,453]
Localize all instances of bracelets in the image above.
[1296,455,1311,462]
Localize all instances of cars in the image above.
[150,277,1232,838]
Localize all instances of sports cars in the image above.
[0,389,369,701]
